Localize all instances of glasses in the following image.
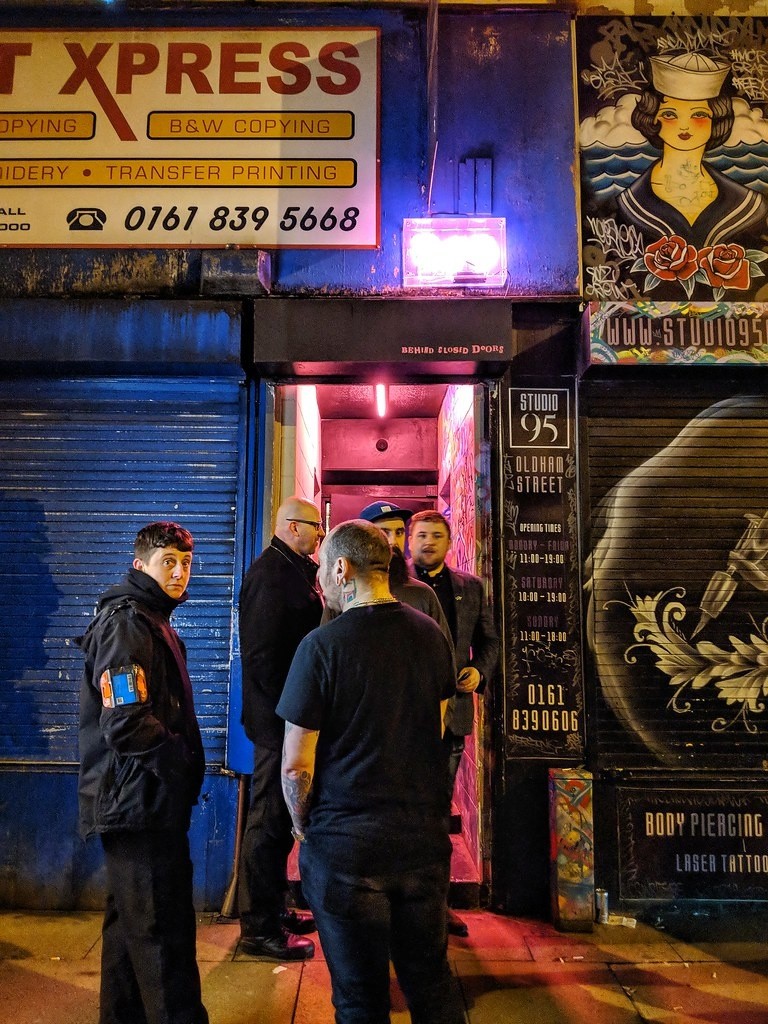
[286,518,323,530]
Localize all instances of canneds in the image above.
[594,888,609,924]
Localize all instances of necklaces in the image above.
[355,598,395,606]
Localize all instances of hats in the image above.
[359,501,413,523]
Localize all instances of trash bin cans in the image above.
[547,767,596,933]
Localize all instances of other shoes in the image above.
[446,906,468,934]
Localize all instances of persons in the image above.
[73,522,210,1024]
[321,500,455,739]
[276,519,457,1024]
[240,497,325,959]
[408,510,500,935]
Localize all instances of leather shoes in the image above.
[240,924,315,962]
[277,907,316,934]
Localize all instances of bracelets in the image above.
[290,827,304,841]
